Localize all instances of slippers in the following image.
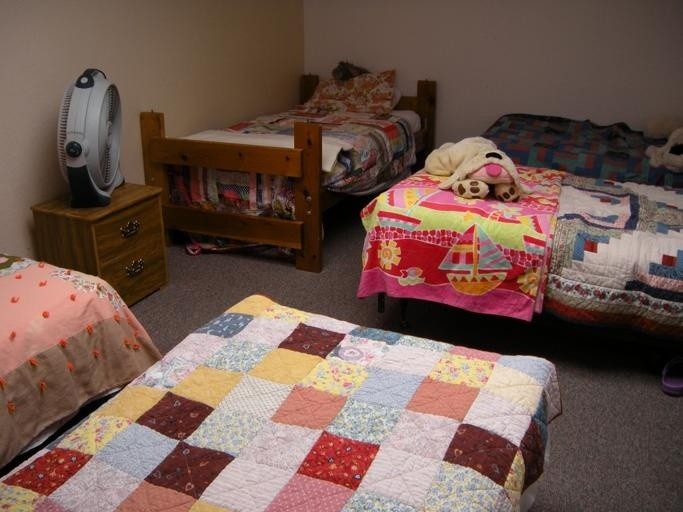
[660,352,683,397]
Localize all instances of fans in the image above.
[56,70,125,208]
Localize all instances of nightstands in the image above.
[30,184,169,307]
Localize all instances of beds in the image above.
[0,293,563,512]
[373,162,683,334]
[140,75,436,273]
[480,113,683,189]
[0,254,163,469]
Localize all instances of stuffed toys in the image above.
[424,136,519,202]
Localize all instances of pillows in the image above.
[303,71,395,114]
